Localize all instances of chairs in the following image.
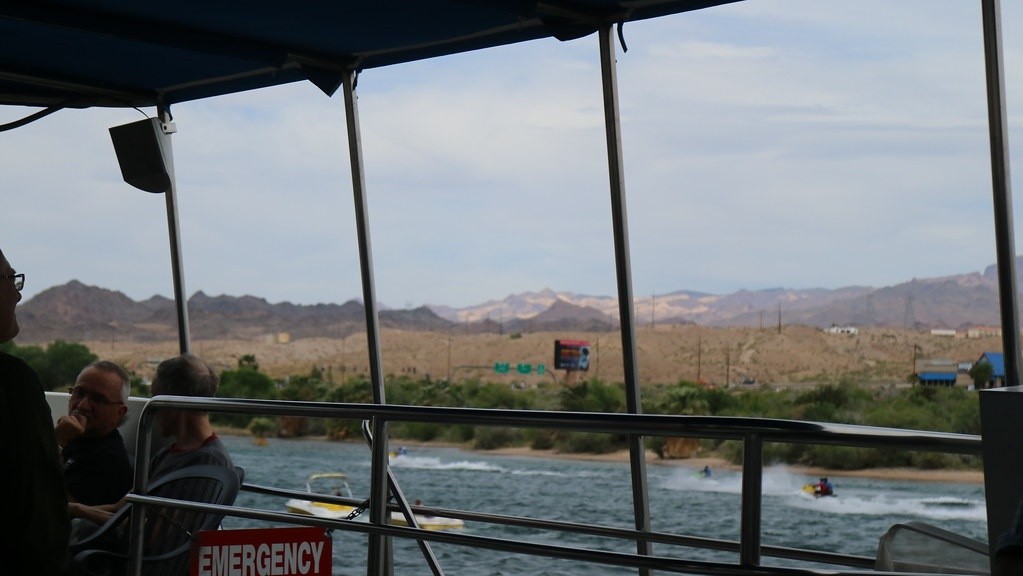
[70,465,239,576]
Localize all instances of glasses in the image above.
[0,274,24,291]
[68,387,123,407]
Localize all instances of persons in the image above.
[415,499,423,505]
[0,248,70,576]
[104,355,240,557]
[57,361,132,515]
[701,466,711,478]
[816,477,833,496]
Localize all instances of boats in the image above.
[801,483,817,499]
[286,492,466,534]
